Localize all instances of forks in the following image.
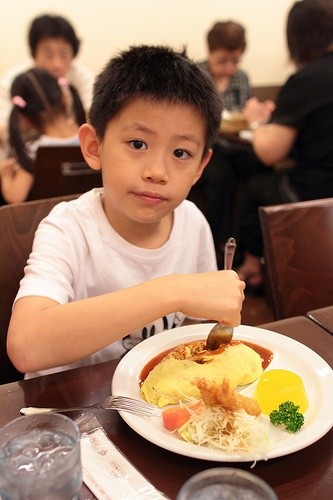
[19,395,156,418]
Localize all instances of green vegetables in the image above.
[270,401,303,433]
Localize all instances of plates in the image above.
[111,320,333,464]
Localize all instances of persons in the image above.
[5,43,245,381]
[185,0,333,294]
[0,15,99,208]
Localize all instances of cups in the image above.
[0,410,83,500]
[175,467,281,500]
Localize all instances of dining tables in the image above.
[0,317,333,500]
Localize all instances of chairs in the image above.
[0,193,82,385]
[258,198,333,321]
[26,146,103,201]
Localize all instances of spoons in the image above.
[205,238,239,351]
